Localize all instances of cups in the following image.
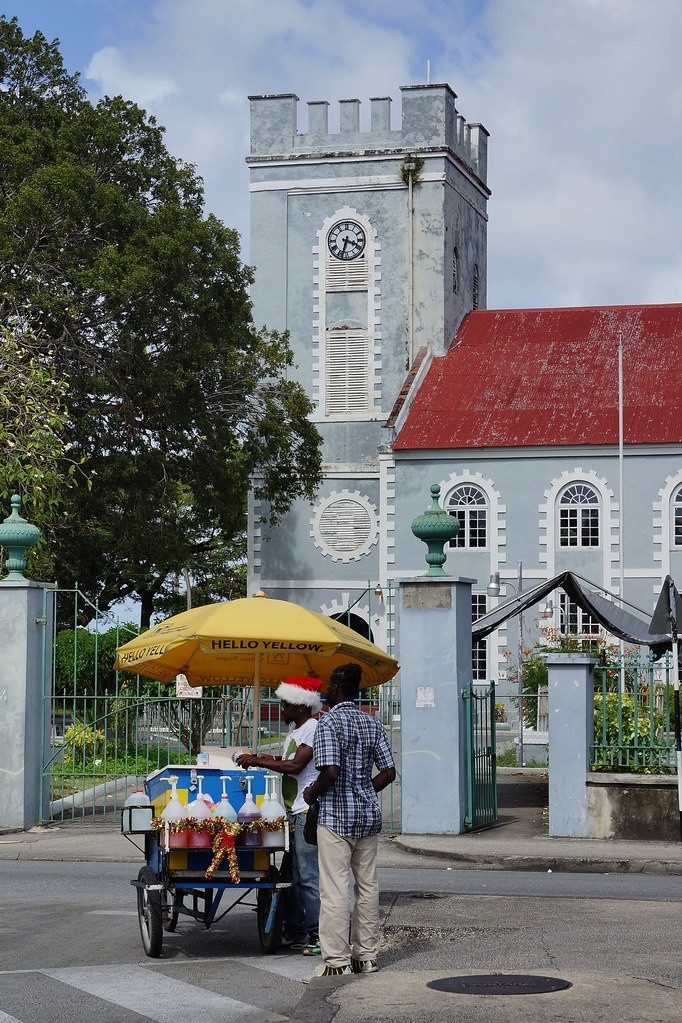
[197,753,210,767]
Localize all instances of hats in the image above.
[275,676,323,715]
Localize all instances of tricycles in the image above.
[121,767,292,956]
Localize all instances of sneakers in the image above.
[351,957,378,972]
[302,965,353,984]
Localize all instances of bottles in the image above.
[123,790,152,832]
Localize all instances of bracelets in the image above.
[273,756,275,760]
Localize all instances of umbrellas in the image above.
[112,593,401,766]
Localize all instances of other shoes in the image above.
[280,933,309,944]
[302,939,322,955]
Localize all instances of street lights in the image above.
[545,597,568,650]
[487,562,523,768]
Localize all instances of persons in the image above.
[233,676,322,956]
[303,663,395,985]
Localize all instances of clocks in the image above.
[326,219,366,262]
